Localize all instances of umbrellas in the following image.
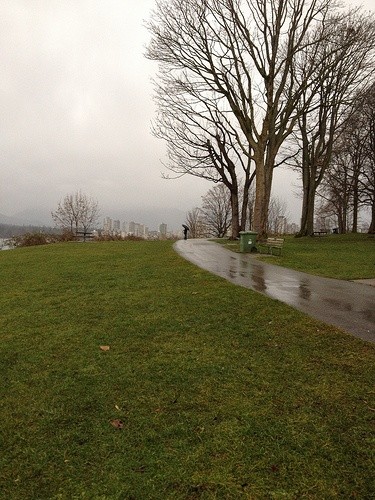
[182,225,189,230]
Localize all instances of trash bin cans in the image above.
[238,230,259,254]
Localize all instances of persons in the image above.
[183,227,188,240]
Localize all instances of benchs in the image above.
[312,229,330,236]
[264,238,285,256]
